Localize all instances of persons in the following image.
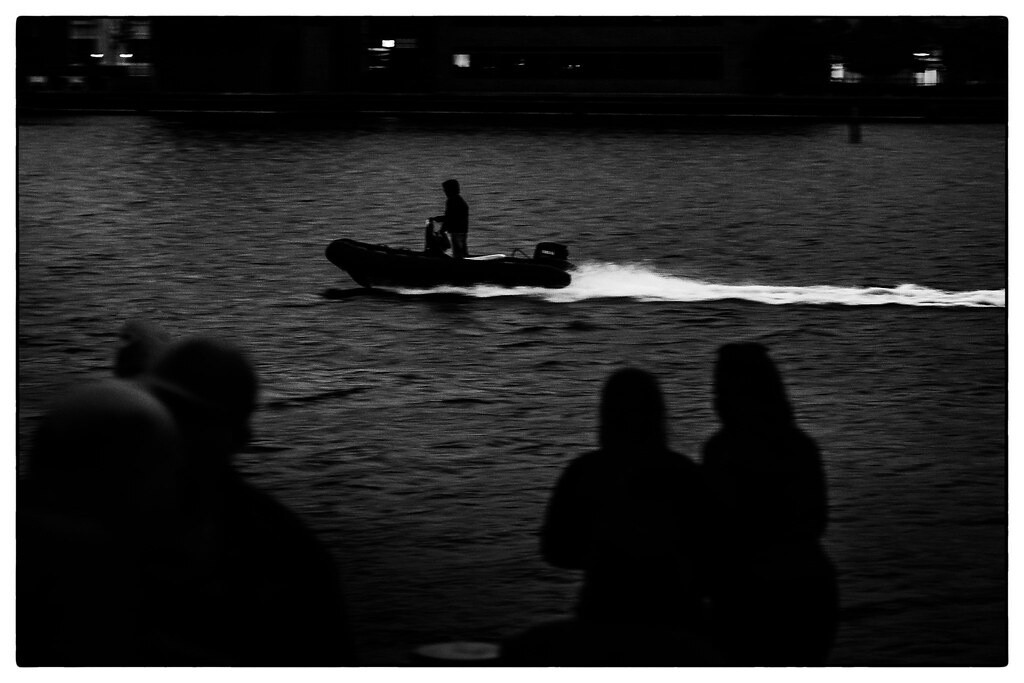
[497,340,840,667]
[16,324,322,666]
[428,179,470,254]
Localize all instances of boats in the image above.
[324,218,574,290]
